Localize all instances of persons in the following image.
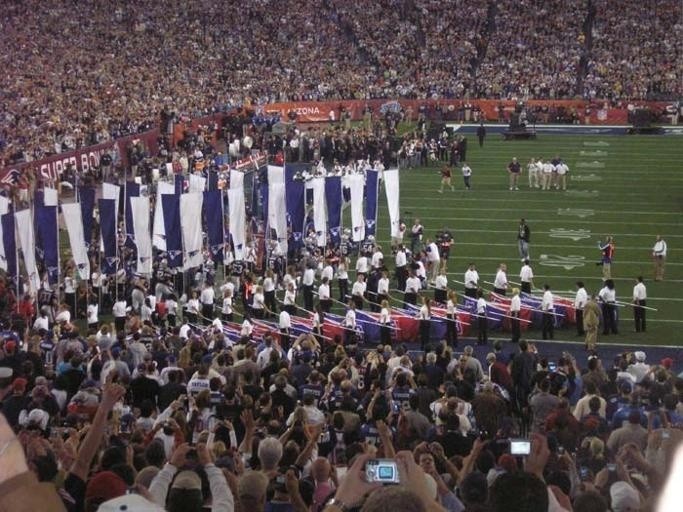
[2,0,683,512]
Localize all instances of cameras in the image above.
[509,438,532,457]
[186,449,197,459]
[364,458,399,484]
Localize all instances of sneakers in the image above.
[510,184,566,191]
[521,257,530,262]
[437,187,471,193]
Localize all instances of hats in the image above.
[0,330,682,512]
